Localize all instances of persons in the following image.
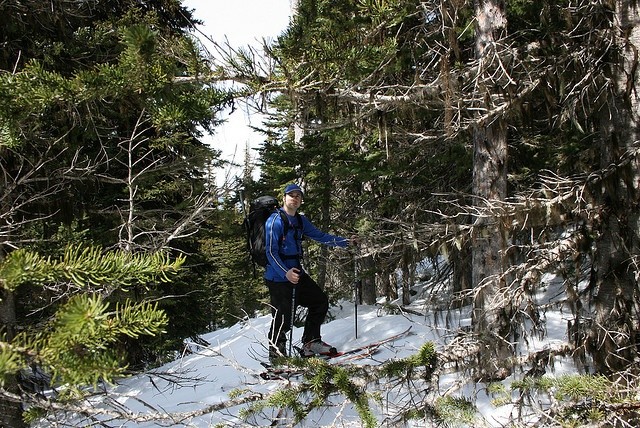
[263,184,358,364]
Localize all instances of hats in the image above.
[285,185,301,194]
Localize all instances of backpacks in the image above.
[247,195,303,268]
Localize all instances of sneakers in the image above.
[302,342,332,356]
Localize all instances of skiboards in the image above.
[261,325,413,380]
